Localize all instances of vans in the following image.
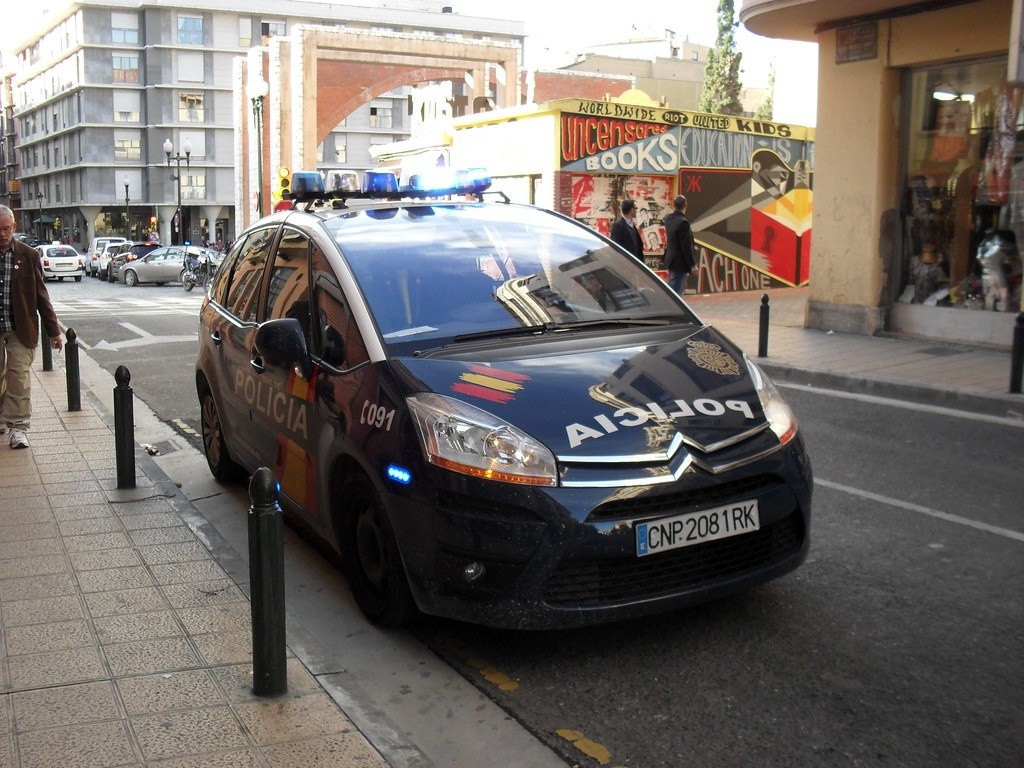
[82,237,127,279]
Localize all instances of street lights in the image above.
[162,137,193,246]
[123,175,132,241]
[37,192,44,245]
[246,75,269,220]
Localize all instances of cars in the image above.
[118,246,227,288]
[194,162,815,632]
[33,245,86,282]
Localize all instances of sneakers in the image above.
[0,423,8,433]
[5,428,29,448]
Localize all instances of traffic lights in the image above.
[271,191,283,205]
[54,219,61,230]
[279,164,292,200]
[147,217,157,235]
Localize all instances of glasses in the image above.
[634,207,637,209]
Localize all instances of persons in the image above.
[200,236,233,254]
[611,200,646,263]
[61,235,74,246]
[661,196,698,299]
[0,204,63,448]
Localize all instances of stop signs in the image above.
[273,199,298,213]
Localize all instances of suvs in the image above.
[95,241,135,281]
[107,240,163,283]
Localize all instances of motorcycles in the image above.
[179,241,219,293]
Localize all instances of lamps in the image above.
[148,216,157,234]
